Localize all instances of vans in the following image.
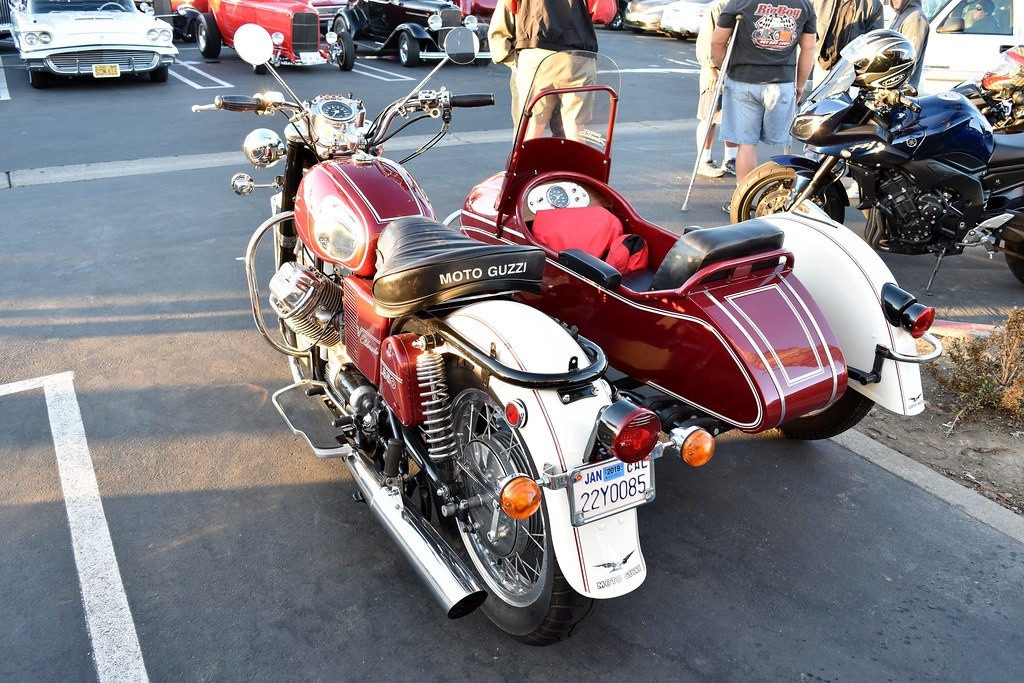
[915,0,1024,98]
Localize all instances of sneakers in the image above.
[698,160,724,178]
[721,160,737,174]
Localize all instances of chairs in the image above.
[620,217,784,293]
[999,8,1011,33]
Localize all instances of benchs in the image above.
[33,3,115,12]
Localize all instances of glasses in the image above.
[977,7,984,11]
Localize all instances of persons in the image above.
[695,0,740,177]
[965,0,998,32]
[890,0,930,89]
[504,0,598,143]
[812,0,885,92]
[488,0,565,141]
[710,0,819,214]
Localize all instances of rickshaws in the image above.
[729,56,1024,297]
[189,22,944,649]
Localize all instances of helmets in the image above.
[981,45,1024,93]
[848,29,914,90]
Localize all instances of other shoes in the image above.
[722,203,750,219]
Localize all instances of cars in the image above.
[8,0,180,89]
[163,0,717,77]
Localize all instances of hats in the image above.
[976,0,994,15]
